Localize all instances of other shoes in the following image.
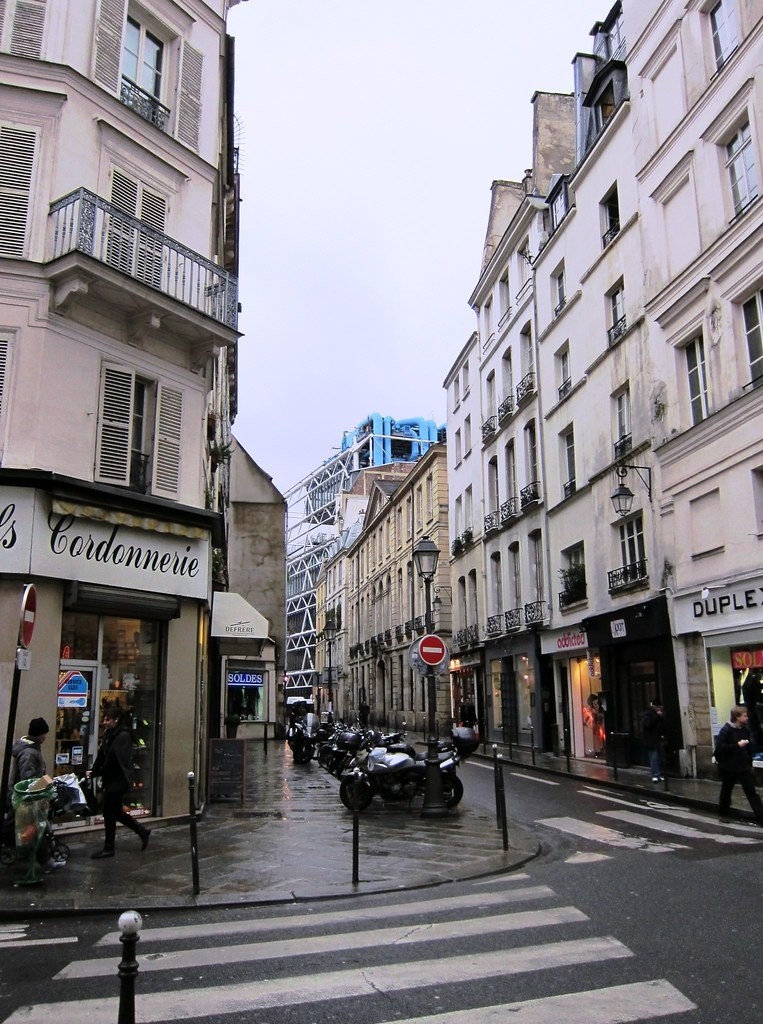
[651,777,659,782]
[660,776,665,780]
[91,849,114,859]
[44,860,66,874]
[139,828,151,851]
[719,816,729,823]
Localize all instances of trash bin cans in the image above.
[11,777,54,885]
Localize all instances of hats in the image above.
[28,717,49,736]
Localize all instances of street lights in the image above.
[411,535,452,817]
[322,618,338,726]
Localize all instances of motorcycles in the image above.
[287,703,320,763]
[315,721,416,777]
[339,719,480,810]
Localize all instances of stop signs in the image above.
[419,635,446,666]
[18,584,39,649]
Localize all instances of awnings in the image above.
[211,591,269,656]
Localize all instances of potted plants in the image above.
[213,548,227,591]
[210,436,236,474]
[556,561,586,604]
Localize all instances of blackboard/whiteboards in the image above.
[207,737,246,796]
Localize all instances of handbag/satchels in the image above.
[53,772,87,812]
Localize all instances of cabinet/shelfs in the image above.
[57,687,150,825]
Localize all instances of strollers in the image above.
[0,775,88,865]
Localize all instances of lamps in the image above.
[701,585,726,598]
[609,459,652,517]
[432,585,453,615]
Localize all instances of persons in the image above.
[11,716,49,863]
[460,695,477,728]
[83,706,153,858]
[643,697,667,781]
[224,709,241,739]
[715,705,763,823]
[359,700,370,728]
